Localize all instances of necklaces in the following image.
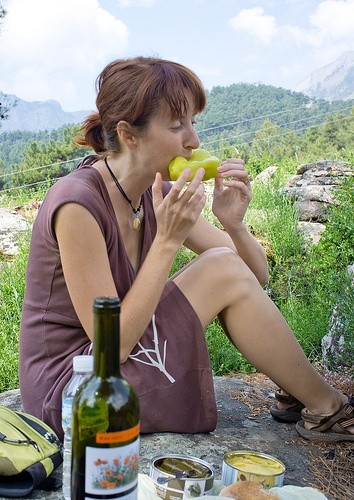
[103,157,145,232]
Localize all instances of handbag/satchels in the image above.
[0,406,63,498]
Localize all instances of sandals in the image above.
[295,394,354,442]
[270,388,305,423]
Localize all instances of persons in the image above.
[16,55,354,449]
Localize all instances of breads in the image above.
[219,479,282,500]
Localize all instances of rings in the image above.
[245,177,250,185]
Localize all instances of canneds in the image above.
[221,450,287,492]
[148,454,216,500]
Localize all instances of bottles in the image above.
[62,355,93,500]
[71,296,141,500]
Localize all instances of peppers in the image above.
[169,149,221,181]
